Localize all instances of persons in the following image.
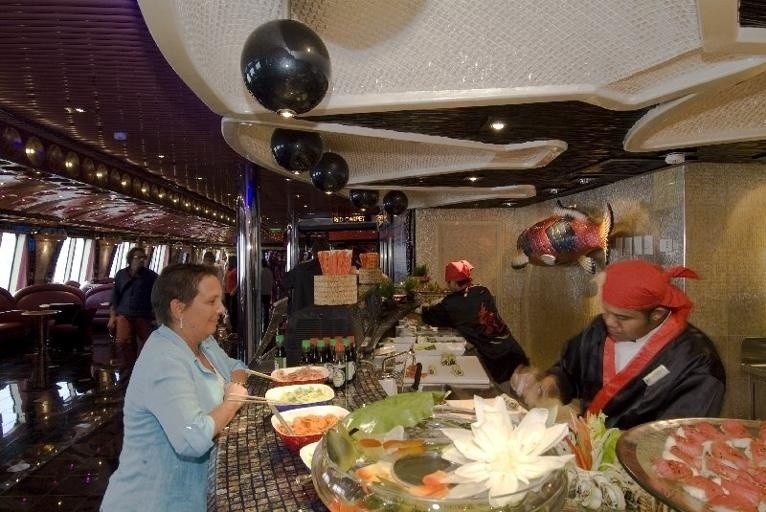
[421,257,531,396]
[509,259,726,428]
[99,262,249,512]
[107,248,158,373]
[204,252,285,341]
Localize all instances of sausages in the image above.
[655,419,766,511]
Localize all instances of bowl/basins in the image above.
[271,404,350,454]
[263,384,335,409]
[270,366,330,384]
[380,320,471,353]
[299,441,317,473]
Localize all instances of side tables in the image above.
[21,309,62,392]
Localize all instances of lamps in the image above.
[310,151,349,194]
[383,190,408,215]
[349,190,379,211]
[270,127,324,175]
[240,0,332,119]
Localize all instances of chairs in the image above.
[0,278,115,352]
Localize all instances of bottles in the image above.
[300,335,358,388]
[273,335,287,369]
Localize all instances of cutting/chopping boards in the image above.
[400,354,492,385]
[445,394,529,417]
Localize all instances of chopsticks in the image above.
[244,368,280,381]
[226,392,290,406]
